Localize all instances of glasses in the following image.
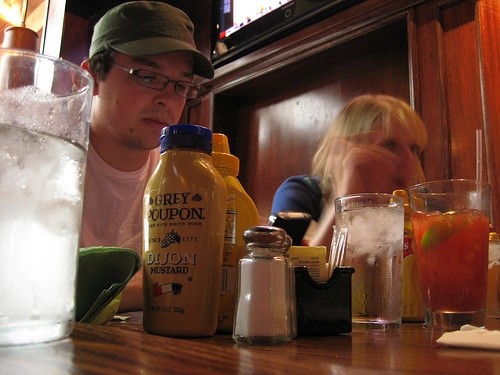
[109,63,202,99]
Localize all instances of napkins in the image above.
[436,324,500,350]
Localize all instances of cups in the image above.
[335,193,405,332]
[407,179,491,335]
[0,46,94,347]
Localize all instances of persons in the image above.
[271,94,431,246]
[2,1,215,314]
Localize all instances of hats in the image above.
[88,2,214,79]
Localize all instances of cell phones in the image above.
[266,213,311,246]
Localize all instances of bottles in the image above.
[485,232,500,331]
[389,191,428,324]
[143,125,228,339]
[232,227,288,345]
[1,25,39,89]
[212,134,260,332]
[284,236,298,341]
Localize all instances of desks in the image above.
[72,310,500,375]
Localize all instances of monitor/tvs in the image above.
[217,0,360,47]
